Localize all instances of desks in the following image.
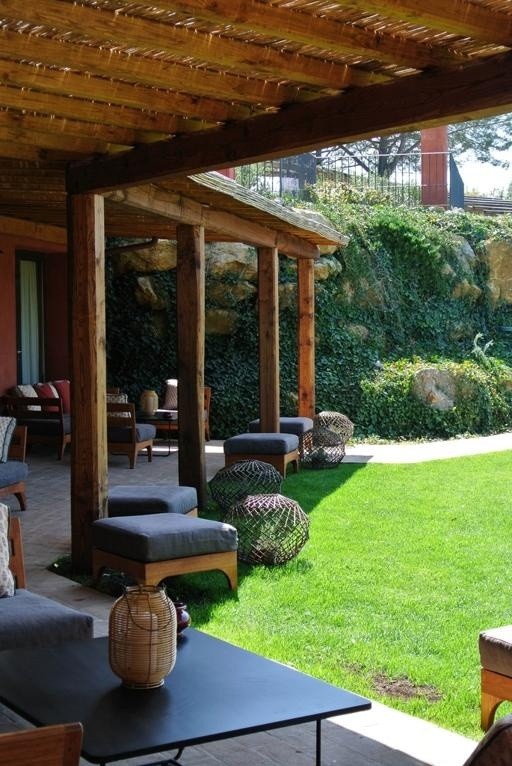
[1,623,372,763]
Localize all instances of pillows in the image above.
[0,501,16,597]
[1,417,18,462]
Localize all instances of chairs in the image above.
[8,375,211,469]
[0,723,85,764]
[0,518,95,654]
[1,423,30,513]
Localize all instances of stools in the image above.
[248,417,315,460]
[94,513,242,596]
[104,484,199,515]
[222,432,301,478]
[474,621,512,736]
